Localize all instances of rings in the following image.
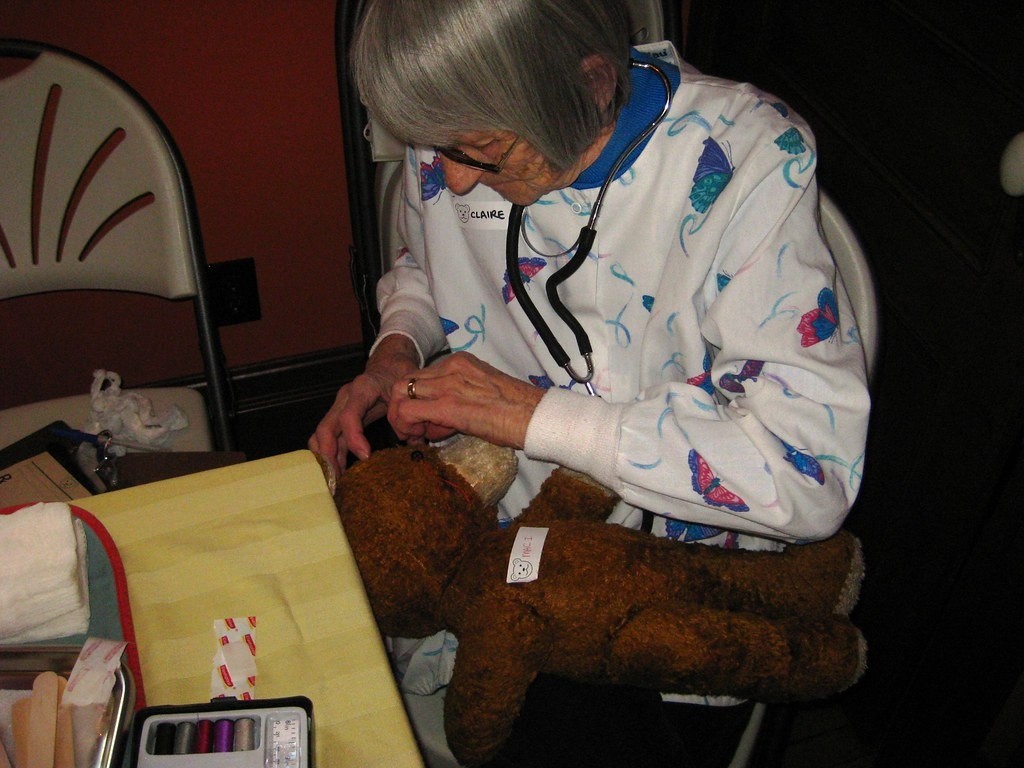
[407,378,417,400]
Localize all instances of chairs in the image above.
[0,37,235,492]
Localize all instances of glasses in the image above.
[435,135,520,174]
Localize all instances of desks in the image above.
[0,450,427,768]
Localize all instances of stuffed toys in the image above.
[322,431,870,766]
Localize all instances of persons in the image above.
[308,0,873,768]
